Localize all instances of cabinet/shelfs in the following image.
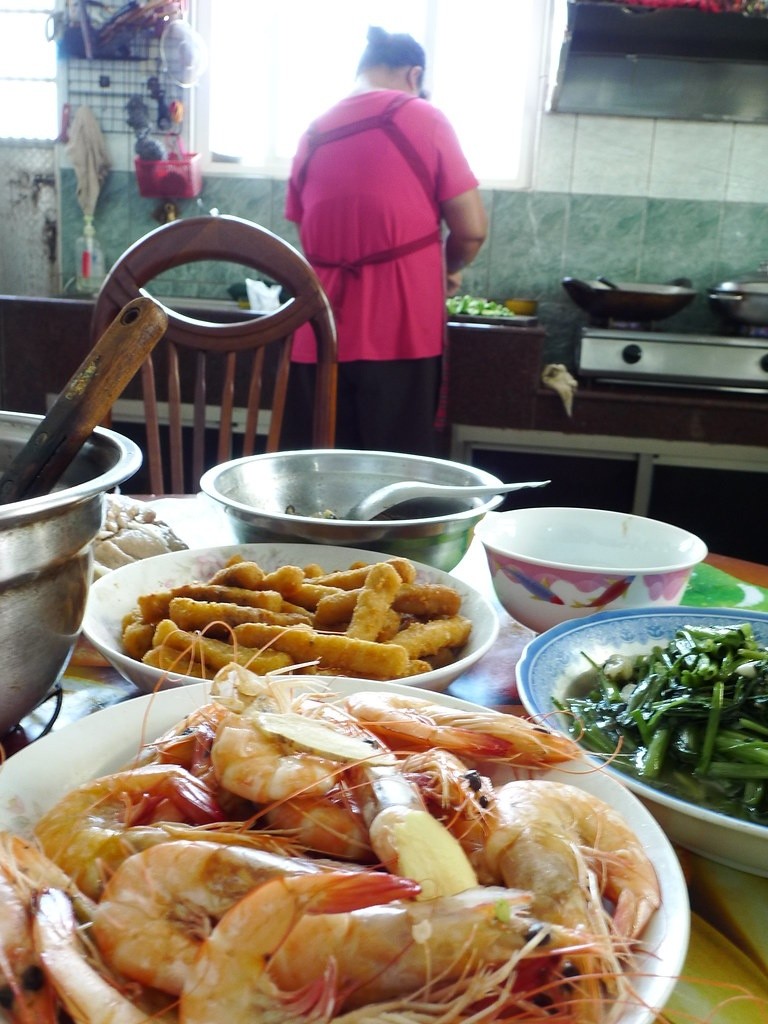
[45,393,768,564]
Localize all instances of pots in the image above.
[707,264,768,328]
[561,276,698,323]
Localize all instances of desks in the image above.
[1,534,768,762]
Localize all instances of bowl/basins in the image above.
[0,409,144,734]
[200,449,508,573]
[474,507,708,633]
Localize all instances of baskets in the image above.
[134,132,203,198]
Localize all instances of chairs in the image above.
[84,213,341,497]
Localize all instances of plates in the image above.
[0,675,691,1024]
[83,542,499,693]
[516,605,768,878]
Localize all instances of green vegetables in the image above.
[551,619,768,829]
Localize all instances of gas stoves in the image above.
[573,317,768,394]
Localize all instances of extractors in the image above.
[544,0,768,124]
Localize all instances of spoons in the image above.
[347,480,552,521]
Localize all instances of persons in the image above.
[281,26,490,459]
